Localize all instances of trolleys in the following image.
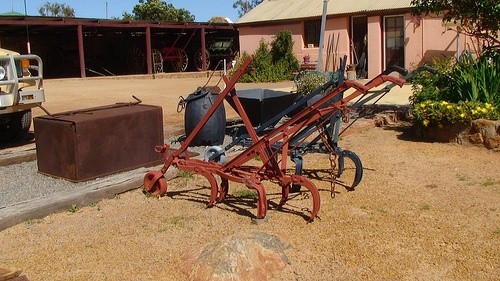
[202,64,437,195]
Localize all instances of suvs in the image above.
[0,48,45,135]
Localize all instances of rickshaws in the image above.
[160,31,195,72]
